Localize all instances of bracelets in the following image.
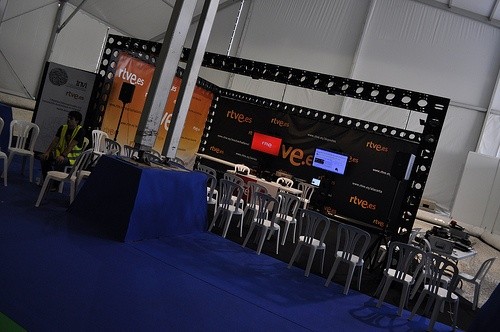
[60,154,65,158]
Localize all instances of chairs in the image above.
[0,119,39,182]
[58,136,89,193]
[365,227,421,269]
[272,193,300,246]
[0,117,8,187]
[194,164,250,239]
[75,151,102,194]
[89,130,109,168]
[272,178,294,211]
[34,148,93,208]
[104,138,162,165]
[287,208,330,278]
[409,239,448,313]
[447,257,497,310]
[325,223,372,295]
[236,181,270,229]
[408,252,459,332]
[242,192,281,257]
[292,183,315,217]
[375,242,427,316]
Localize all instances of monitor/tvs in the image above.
[249,129,284,160]
[311,146,350,176]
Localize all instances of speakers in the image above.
[118,82,136,104]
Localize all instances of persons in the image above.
[38,111,84,191]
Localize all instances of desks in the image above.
[227,170,302,211]
[66,155,208,242]
[451,248,477,259]
